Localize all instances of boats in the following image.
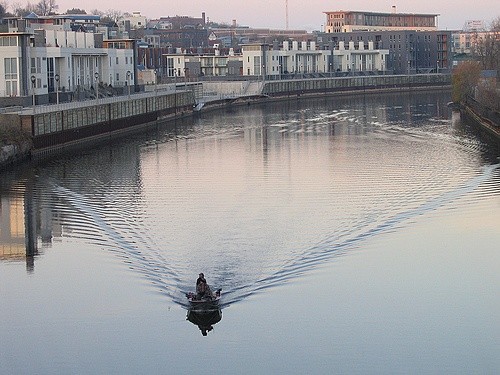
[186,310,223,336]
[186,296,222,312]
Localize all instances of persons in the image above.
[200,279,213,300]
[196,273,206,300]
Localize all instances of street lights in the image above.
[262,60,442,83]
[30,68,178,106]
